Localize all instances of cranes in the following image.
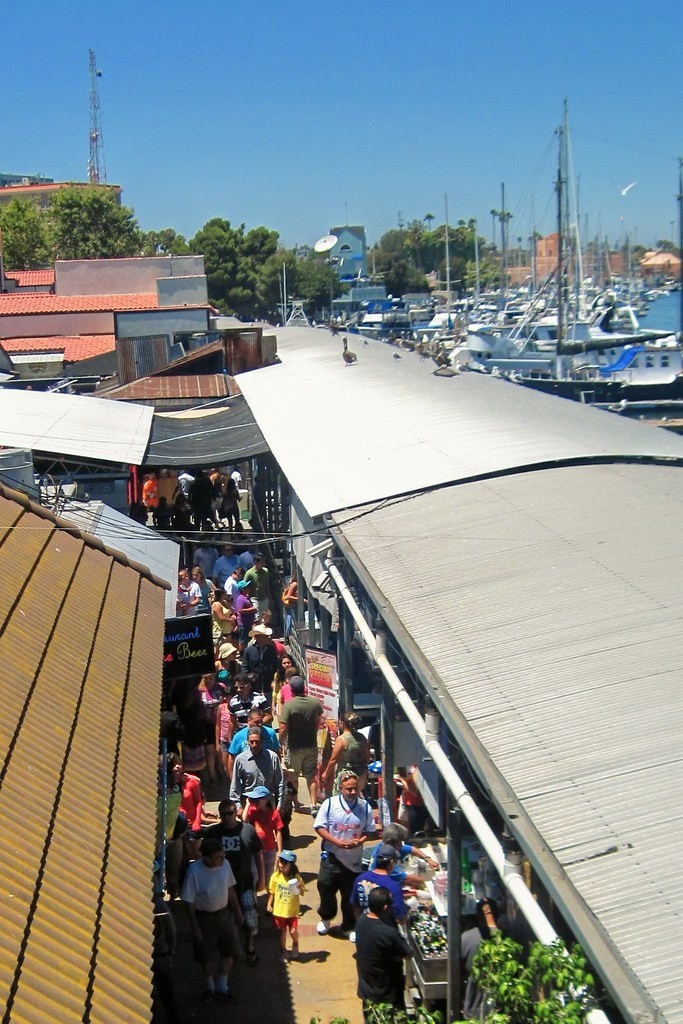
[86,47,107,184]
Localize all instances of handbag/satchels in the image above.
[281,583,298,609]
[204,579,216,607]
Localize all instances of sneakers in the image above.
[288,945,300,958]
[292,801,300,811]
[280,950,289,964]
[343,930,356,942]
[310,803,318,815]
[317,919,330,935]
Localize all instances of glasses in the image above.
[341,775,357,784]
[224,548,233,552]
[233,683,251,690]
[219,807,236,817]
[221,591,227,595]
[213,851,227,859]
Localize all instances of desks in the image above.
[395,922,449,1014]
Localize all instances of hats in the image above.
[248,624,273,637]
[236,580,252,592]
[278,849,297,863]
[218,642,237,659]
[289,672,304,690]
[376,844,401,862]
[199,838,223,857]
[242,786,271,798]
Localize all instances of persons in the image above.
[136,465,537,1024]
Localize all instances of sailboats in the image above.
[275,93,683,432]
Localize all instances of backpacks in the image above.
[218,503,227,519]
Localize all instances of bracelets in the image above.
[424,856,430,862]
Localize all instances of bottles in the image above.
[408,887,448,961]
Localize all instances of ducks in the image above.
[342,336,357,366]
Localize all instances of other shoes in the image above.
[199,984,216,1005]
[215,989,240,1002]
[245,949,259,964]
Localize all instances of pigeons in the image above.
[608,399,628,416]
[359,338,436,363]
